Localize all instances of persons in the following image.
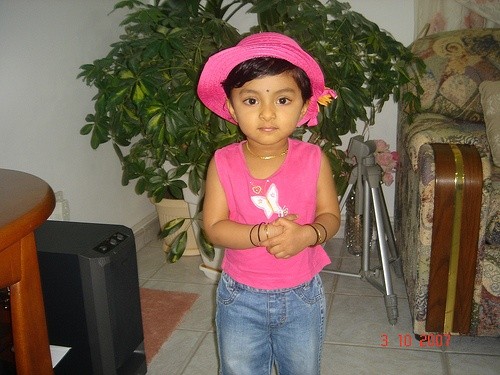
[197,32,341,375]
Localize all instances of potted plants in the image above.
[77,0,438,271]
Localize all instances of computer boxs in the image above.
[0,219,147,375]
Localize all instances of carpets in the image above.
[139,286,200,369]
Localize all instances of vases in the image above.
[346,194,374,254]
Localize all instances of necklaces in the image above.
[245,141,288,160]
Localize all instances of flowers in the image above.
[338,139,398,186]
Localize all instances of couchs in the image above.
[394,27,500,342]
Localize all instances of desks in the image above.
[0,168,57,375]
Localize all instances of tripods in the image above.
[314,135,403,330]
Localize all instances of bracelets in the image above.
[306,223,327,248]
[249,222,269,248]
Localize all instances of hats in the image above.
[196,32,338,128]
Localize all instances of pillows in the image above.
[478,80,500,166]
